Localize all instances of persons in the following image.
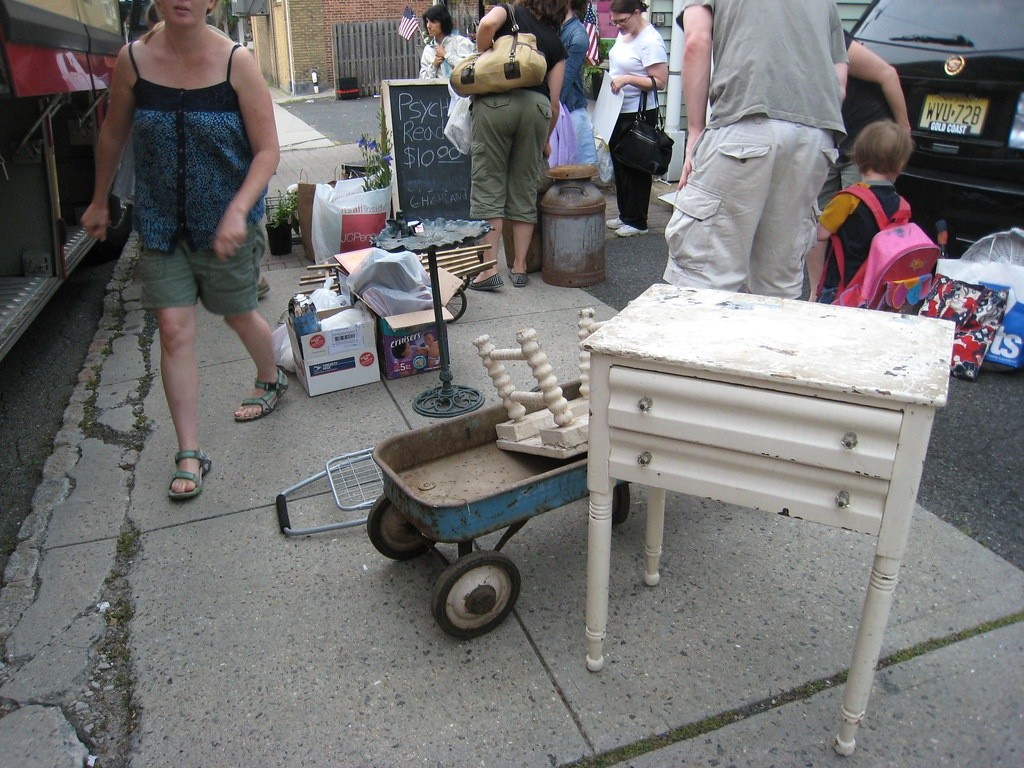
[657,0,848,300]
[82,0,289,500]
[606,0,668,236]
[417,4,474,79]
[557,0,599,163]
[469,0,569,291]
[843,28,911,134]
[816,120,913,304]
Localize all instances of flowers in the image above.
[347,107,393,192]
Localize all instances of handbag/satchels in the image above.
[311,166,394,264]
[612,75,675,175]
[450,4,548,98]
[936,237,1024,369]
[444,96,473,155]
[916,273,1008,381]
[297,166,341,263]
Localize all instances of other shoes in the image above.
[468,272,504,290]
[508,270,528,287]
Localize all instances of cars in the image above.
[841,0,1024,250]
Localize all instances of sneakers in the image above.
[615,225,648,237]
[606,217,624,229]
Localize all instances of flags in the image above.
[582,4,599,67]
[399,5,420,41]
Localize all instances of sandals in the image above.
[234,367,289,422]
[168,447,211,499]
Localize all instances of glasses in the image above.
[608,8,641,26]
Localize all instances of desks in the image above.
[578,283,956,759]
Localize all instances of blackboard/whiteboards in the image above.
[381,80,486,224]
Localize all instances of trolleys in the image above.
[364,374,634,640]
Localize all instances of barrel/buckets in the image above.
[542,164,608,287]
[336,76,359,100]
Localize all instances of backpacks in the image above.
[816,185,941,313]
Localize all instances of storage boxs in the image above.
[285,304,382,398]
[334,247,465,380]
[917,236,1024,380]
[295,165,344,262]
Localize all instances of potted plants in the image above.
[262,189,301,256]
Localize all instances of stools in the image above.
[474,302,636,460]
